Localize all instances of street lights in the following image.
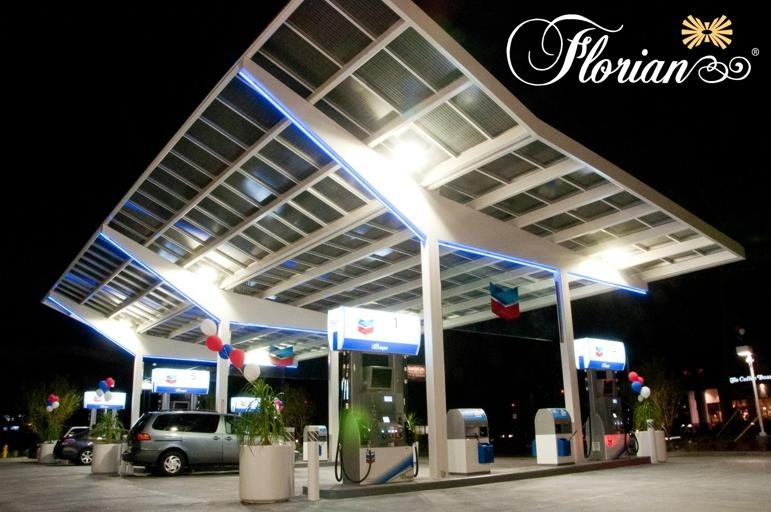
[735,344,766,437]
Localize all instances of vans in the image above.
[53,424,130,465]
[122,411,300,478]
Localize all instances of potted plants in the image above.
[224,375,296,504]
[28,380,83,464]
[406,410,420,465]
[90,410,125,473]
[633,397,667,463]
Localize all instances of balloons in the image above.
[274,397,285,418]
[200,318,261,384]
[47,394,60,412]
[629,372,651,402]
[97,377,116,401]
